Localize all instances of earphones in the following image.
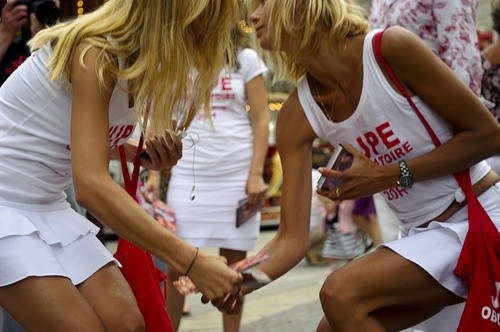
[189,185,196,200]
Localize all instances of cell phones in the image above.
[139,131,188,160]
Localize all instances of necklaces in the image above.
[314,37,348,122]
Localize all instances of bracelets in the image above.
[184,247,199,277]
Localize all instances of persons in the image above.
[201,0,500,332]
[368,0,500,177]
[0,0,107,87]
[313,190,385,270]
[0,0,256,332]
[142,25,271,332]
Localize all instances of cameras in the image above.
[13,0,60,26]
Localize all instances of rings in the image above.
[335,187,340,198]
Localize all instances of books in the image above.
[317,144,353,191]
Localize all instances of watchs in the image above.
[398,160,415,189]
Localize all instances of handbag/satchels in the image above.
[454,196,500,332]
[110,236,174,332]
